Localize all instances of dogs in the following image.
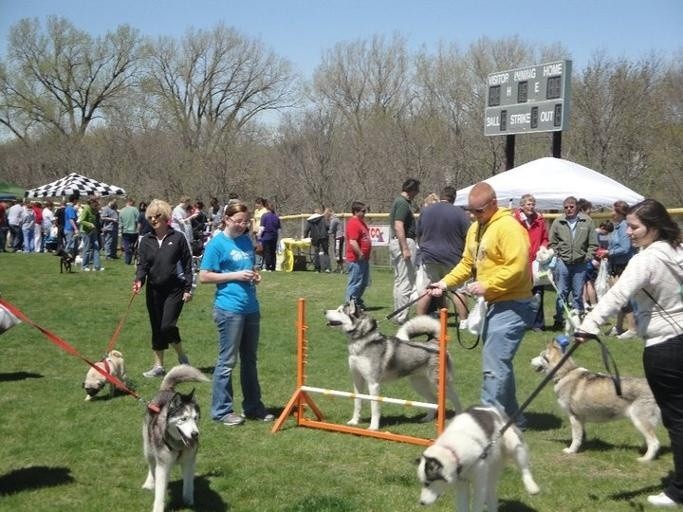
[409,401,543,511]
[323,295,464,432]
[79,349,128,402]
[56,248,73,274]
[528,335,663,464]
[139,362,214,512]
[565,308,588,336]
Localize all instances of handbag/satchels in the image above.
[532,260,553,286]
[594,257,612,303]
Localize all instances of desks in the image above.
[280,237,316,271]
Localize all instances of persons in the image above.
[388,179,421,325]
[513,193,548,332]
[577,198,641,338]
[304,202,371,310]
[198,199,274,424]
[549,196,599,332]
[417,186,471,330]
[426,182,537,432]
[574,199,683,507]
[133,198,193,377]
[0,192,282,272]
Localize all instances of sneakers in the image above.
[647,492,677,505]
[143,366,164,377]
[241,410,274,422]
[531,305,637,339]
[220,412,245,425]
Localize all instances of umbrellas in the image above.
[24,171,128,203]
[452,158,644,209]
